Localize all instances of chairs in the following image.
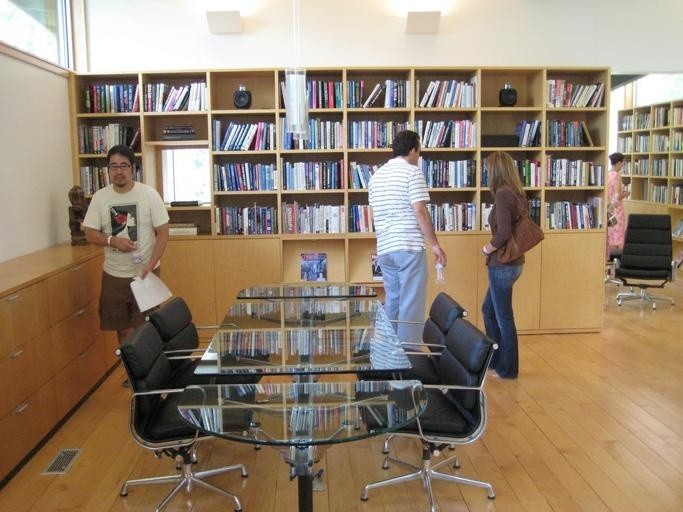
[359,317,498,512]
[358,292,467,455]
[149,296,269,451]
[119,322,250,511]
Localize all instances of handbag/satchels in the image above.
[491,185,545,263]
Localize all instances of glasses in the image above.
[106,163,132,170]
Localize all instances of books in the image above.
[279,157,343,191]
[620,104,683,127]
[78,122,139,153]
[618,134,683,154]
[163,124,197,134]
[545,78,606,110]
[280,79,344,109]
[547,119,595,147]
[484,158,487,188]
[224,382,395,401]
[426,202,476,233]
[214,202,277,235]
[348,78,406,108]
[281,202,345,235]
[350,119,410,149]
[80,165,110,196]
[544,197,602,231]
[643,179,683,205]
[83,82,139,112]
[213,162,278,192]
[222,364,420,378]
[527,196,540,226]
[516,159,540,189]
[351,204,375,234]
[415,119,478,149]
[481,202,495,231]
[143,82,207,113]
[213,119,276,151]
[170,229,199,237]
[288,400,405,438]
[135,163,143,182]
[349,163,381,188]
[171,201,199,207]
[419,157,476,189]
[180,406,226,436]
[622,158,683,178]
[213,326,372,359]
[546,153,603,189]
[169,222,196,227]
[518,117,540,147]
[279,117,344,149]
[416,78,477,110]
[228,285,384,315]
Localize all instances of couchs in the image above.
[607,213,676,309]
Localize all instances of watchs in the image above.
[107,233,113,250]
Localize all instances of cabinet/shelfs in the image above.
[616,100,683,259]
[0,241,114,480]
[71,68,611,335]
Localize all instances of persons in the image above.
[607,153,630,276]
[482,151,531,380]
[82,145,170,389]
[367,130,447,352]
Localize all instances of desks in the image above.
[180,286,427,511]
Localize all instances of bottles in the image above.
[433,258,446,284]
[132,245,143,276]
